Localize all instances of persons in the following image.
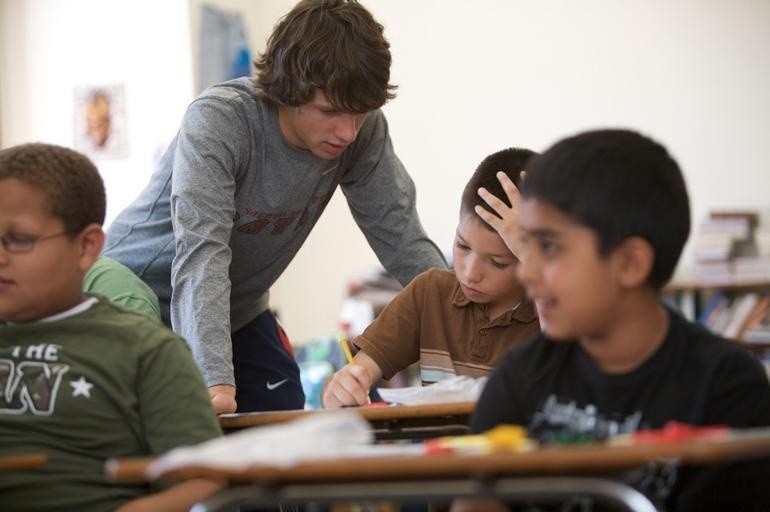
[81,254,162,325]
[447,129,770,512]
[0,142,230,511]
[321,149,542,410]
[93,0,451,416]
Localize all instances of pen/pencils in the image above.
[339,335,371,405]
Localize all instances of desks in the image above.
[0,402,770,512]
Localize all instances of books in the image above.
[655,212,770,380]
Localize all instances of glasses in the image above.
[0,228,82,254]
